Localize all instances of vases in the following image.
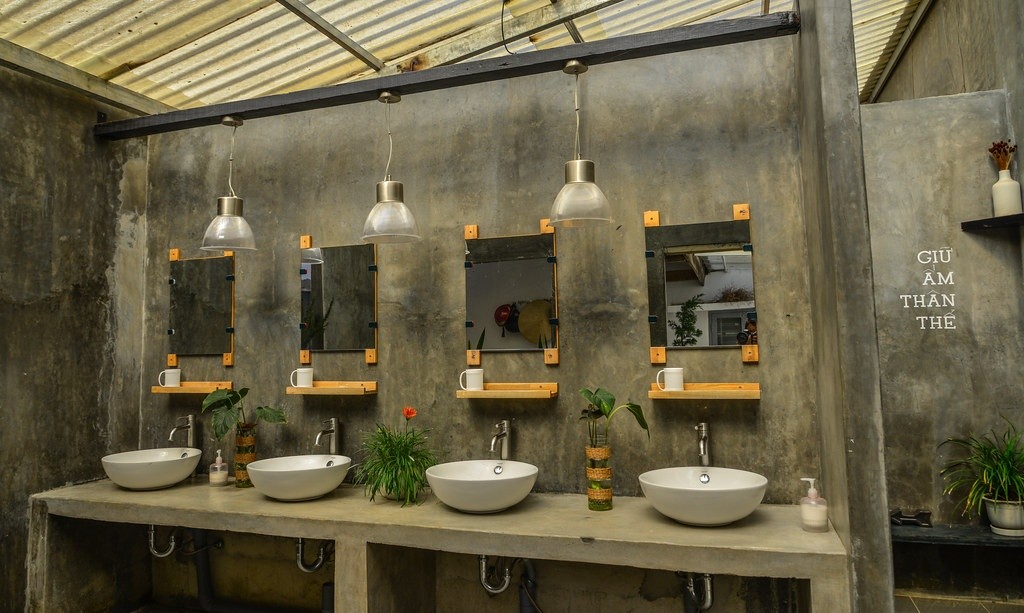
[585,435,613,511]
[235,424,256,488]
[380,471,421,499]
[992,170,1022,217]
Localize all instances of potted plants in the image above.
[935,413,1024,530]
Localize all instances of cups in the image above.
[460,369,484,391]
[290,368,313,387]
[657,367,684,390]
[158,369,180,387]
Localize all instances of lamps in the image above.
[200,116,257,252]
[548,59,613,227]
[360,91,420,245]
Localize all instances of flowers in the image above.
[988,139,1017,171]
[346,404,438,507]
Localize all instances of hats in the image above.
[518,299,552,342]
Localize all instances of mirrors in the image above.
[466,233,557,351]
[300,244,377,352]
[645,220,758,348]
[170,256,234,356]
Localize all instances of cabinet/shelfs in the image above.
[890,523,1024,612]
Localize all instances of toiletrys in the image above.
[800,476,830,532]
[209,449,229,487]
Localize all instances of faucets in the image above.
[314,418,339,455]
[167,414,198,478]
[488,419,513,461]
[693,422,714,468]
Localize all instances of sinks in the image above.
[245,454,351,502]
[100,447,203,491]
[426,459,539,514]
[638,466,769,527]
